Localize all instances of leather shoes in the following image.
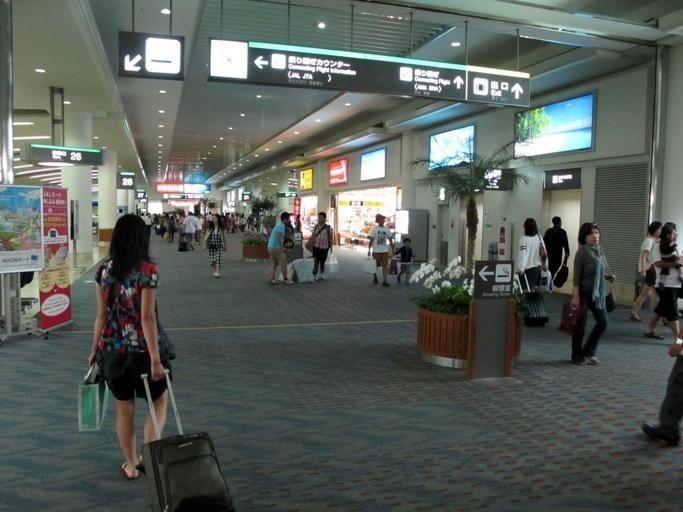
[643,423,680,446]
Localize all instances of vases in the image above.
[243,245,270,262]
[416,307,522,369]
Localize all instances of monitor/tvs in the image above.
[511,87,599,156]
[427,121,478,172]
[360,144,388,181]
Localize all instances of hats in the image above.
[375,214,385,223]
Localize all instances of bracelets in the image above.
[152,360,161,364]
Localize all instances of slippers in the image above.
[122,462,139,480]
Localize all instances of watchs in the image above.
[675,337,683,345]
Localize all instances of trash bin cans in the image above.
[286,231,303,263]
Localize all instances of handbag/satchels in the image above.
[76,375,109,431]
[558,301,586,334]
[362,256,377,274]
[389,259,398,274]
[606,292,615,312]
[553,265,568,288]
[305,239,314,252]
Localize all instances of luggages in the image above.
[140,368,234,512]
[516,271,549,326]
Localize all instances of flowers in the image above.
[409,255,533,319]
[240,231,270,249]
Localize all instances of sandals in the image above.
[630,312,642,321]
[644,331,664,340]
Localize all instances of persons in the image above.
[543,217,569,287]
[368,214,396,287]
[116,204,254,279]
[571,222,615,365]
[519,218,547,292]
[642,327,683,446]
[311,212,333,281]
[267,212,295,285]
[88,213,175,480]
[629,221,683,339]
[394,238,415,285]
